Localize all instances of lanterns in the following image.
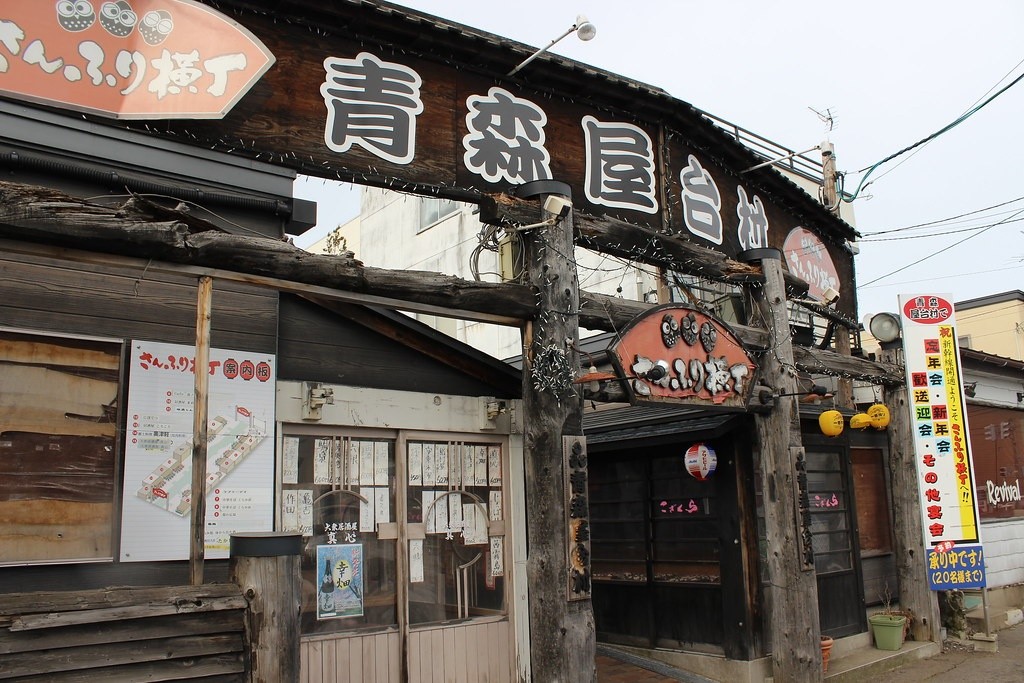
[850,413,872,428]
[867,404,890,429]
[819,410,844,438]
[685,443,717,481]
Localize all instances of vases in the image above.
[821,635,833,672]
[869,614,906,651]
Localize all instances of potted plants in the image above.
[867,581,912,645]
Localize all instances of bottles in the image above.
[321,560,335,614]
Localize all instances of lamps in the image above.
[568,339,616,392]
[868,312,904,343]
[502,195,573,236]
[736,140,833,174]
[508,15,595,76]
[602,361,670,388]
[965,382,977,398]
[788,287,840,306]
[758,384,829,404]
